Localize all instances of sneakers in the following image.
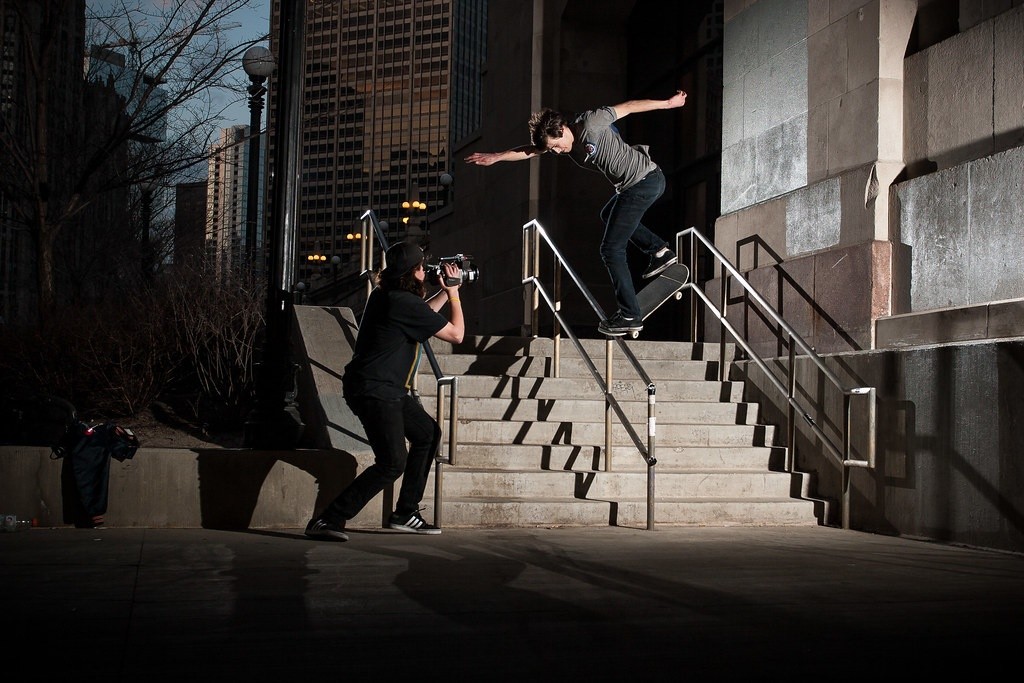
[389,506,443,535]
[642,251,676,279]
[305,516,349,542]
[599,309,644,332]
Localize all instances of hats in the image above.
[383,241,423,278]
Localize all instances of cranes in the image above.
[98,20,242,71]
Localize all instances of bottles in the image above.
[1,514,38,530]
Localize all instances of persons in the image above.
[301,242,466,543]
[462,89,688,333]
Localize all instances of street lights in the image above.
[403,200,427,218]
[379,221,389,251]
[439,173,453,207]
[348,233,367,256]
[296,281,305,305]
[240,45,275,299]
[332,256,341,283]
[308,254,327,275]
[138,172,158,252]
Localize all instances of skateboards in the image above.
[596,262,691,338]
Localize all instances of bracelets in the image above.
[447,297,461,303]
